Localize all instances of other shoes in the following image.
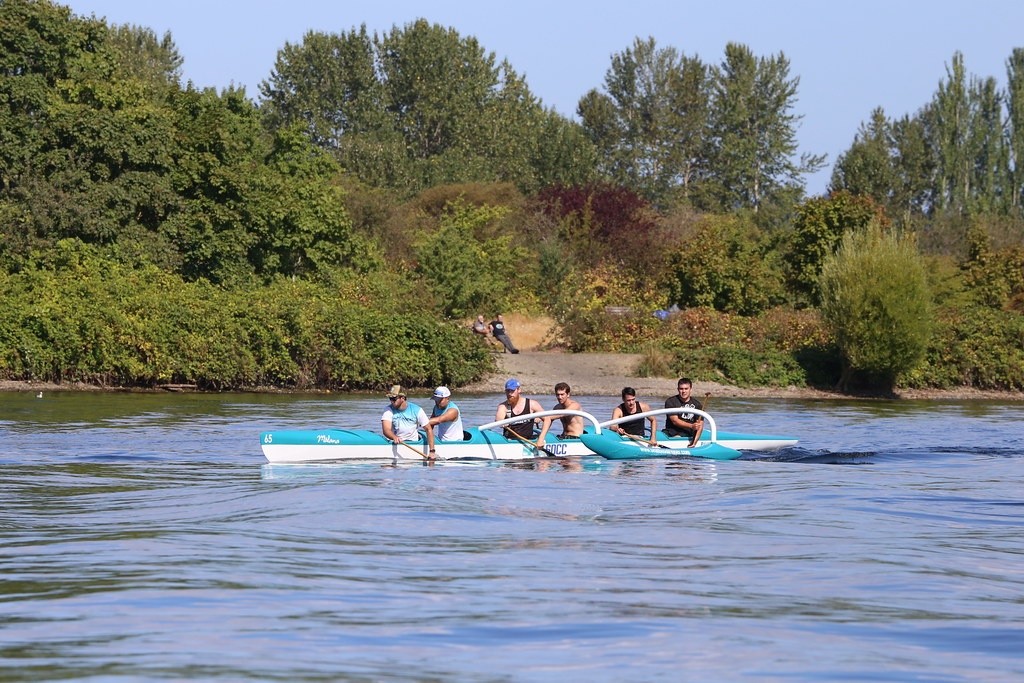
[511,349,519,353]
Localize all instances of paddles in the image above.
[687,392,711,449]
[506,426,555,457]
[621,431,671,449]
[399,440,429,459]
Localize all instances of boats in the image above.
[579,433,743,462]
[257,408,802,466]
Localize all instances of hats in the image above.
[505,379,520,391]
[430,386,451,401]
[386,385,407,397]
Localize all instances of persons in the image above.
[474,315,498,346]
[495,379,551,449]
[610,387,657,447]
[488,313,519,355]
[381,385,437,461]
[534,382,584,439]
[661,378,704,448]
[428,386,464,442]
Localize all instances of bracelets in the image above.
[430,449,435,452]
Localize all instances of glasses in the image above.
[389,397,399,401]
